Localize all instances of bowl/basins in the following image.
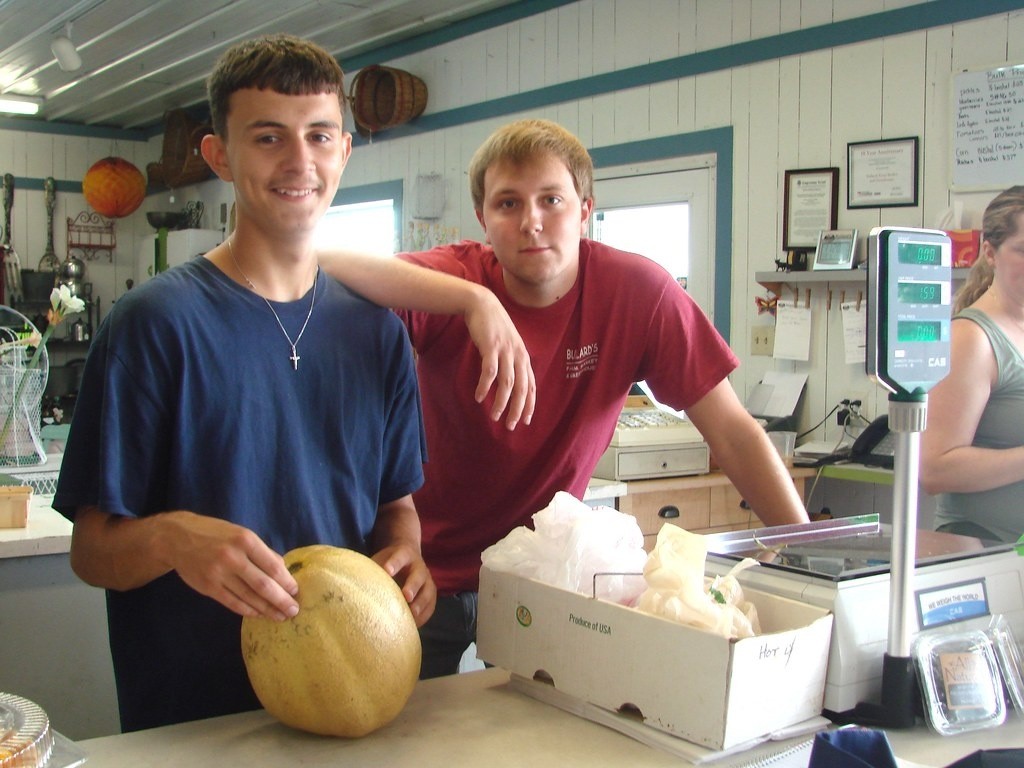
[146,211,181,228]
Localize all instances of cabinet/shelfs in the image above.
[618,468,817,553]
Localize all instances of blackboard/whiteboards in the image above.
[947,59,1024,193]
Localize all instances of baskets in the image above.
[145,107,215,189]
[348,65,428,134]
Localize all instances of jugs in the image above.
[69,320,90,342]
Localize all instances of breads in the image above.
[0,726,39,768]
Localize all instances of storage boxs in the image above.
[766,429,797,469]
[476,562,834,750]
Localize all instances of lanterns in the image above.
[82,157,146,218]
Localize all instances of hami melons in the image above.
[242,544,422,737]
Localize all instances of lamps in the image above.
[50,20,82,72]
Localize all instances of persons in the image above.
[918,184,1023,549]
[313,117,811,679]
[52,37,439,733]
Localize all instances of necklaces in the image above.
[226,236,316,370]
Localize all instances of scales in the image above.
[686,227,1024,727]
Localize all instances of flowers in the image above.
[1,284,88,452]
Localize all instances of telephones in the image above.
[850,414,895,470]
